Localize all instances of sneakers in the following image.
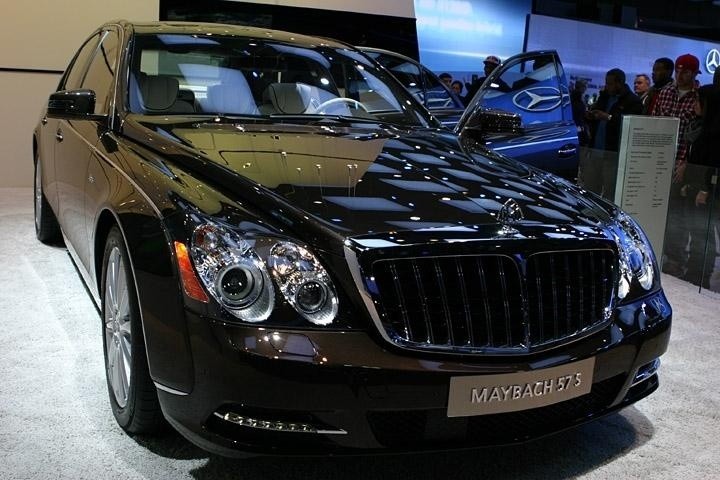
[666,259,681,275]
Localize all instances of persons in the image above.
[569,54,720,289]
[440,54,553,109]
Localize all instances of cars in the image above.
[32,20,672,459]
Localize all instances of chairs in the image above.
[257,83,319,114]
[133,76,194,113]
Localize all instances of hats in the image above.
[676,54,702,74]
[576,79,586,88]
[483,55,501,65]
